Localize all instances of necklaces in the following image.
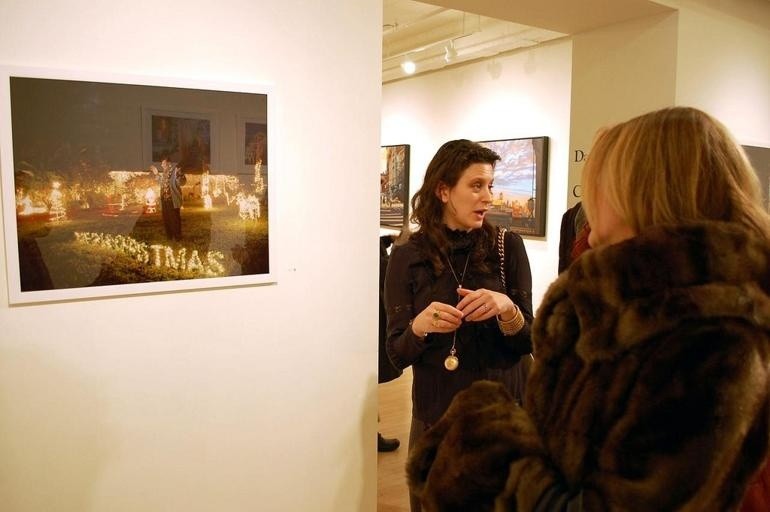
[430,239,479,370]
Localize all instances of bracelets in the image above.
[496,305,526,337]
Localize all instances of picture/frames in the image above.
[0,66,281,309]
[379,144,411,231]
[140,105,267,177]
[478,136,549,237]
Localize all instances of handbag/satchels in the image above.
[558,201,582,275]
[481,353,535,405]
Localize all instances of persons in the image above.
[409,109,769,512]
[386,141,536,458]
[558,199,593,278]
[150,158,185,240]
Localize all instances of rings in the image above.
[483,303,488,312]
[431,321,437,328]
[432,309,440,319]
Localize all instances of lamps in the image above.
[398,41,461,75]
